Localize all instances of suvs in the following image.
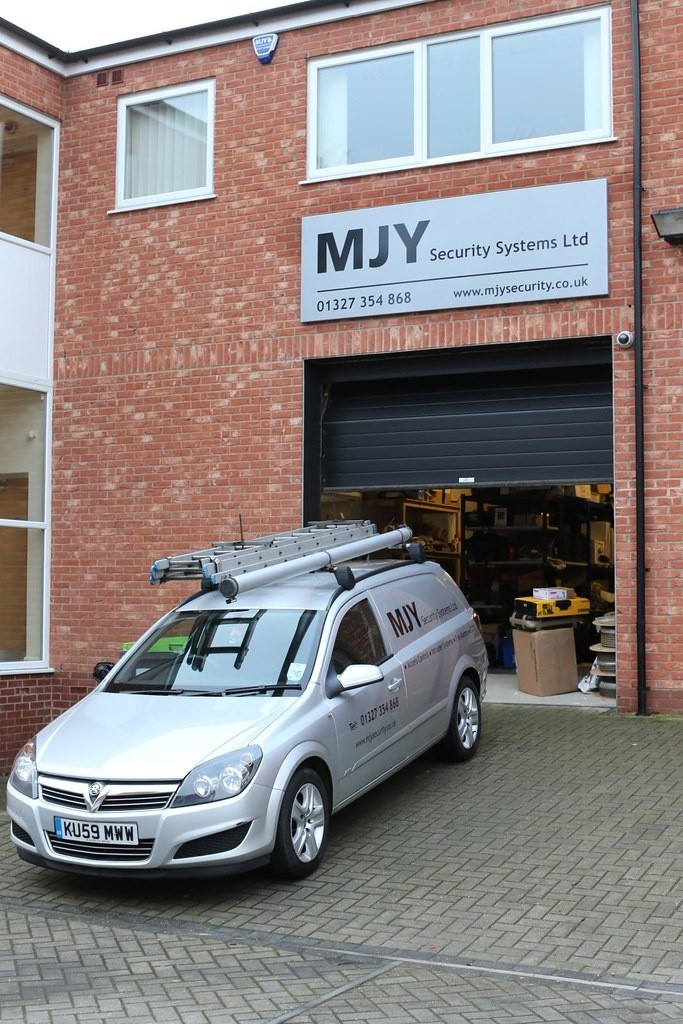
[5,544,491,881]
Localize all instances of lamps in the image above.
[650,208,682,245]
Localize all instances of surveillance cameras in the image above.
[617,331,634,348]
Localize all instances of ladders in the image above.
[150,518,379,584]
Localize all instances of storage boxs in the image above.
[465,511,489,527]
[513,596,590,618]
[464,535,503,565]
[509,612,582,632]
[511,628,578,697]
[533,587,576,599]
[471,571,493,601]
[566,484,613,502]
[589,539,606,564]
[489,507,511,526]
[502,569,543,594]
[563,531,588,562]
[513,512,549,527]
[481,623,504,656]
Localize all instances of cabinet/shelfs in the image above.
[363,500,460,586]
[460,493,616,664]
[321,491,360,519]
